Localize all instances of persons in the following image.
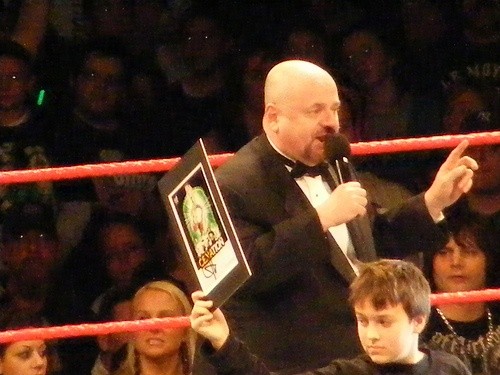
[0,0,500,319]
[125,282,214,375]
[213,59,480,371]
[0,311,50,375]
[190,258,471,375]
[0,200,101,375]
[417,210,500,374]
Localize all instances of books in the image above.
[158,139,253,315]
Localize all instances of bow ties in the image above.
[278,153,329,178]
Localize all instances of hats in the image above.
[12,198,56,229]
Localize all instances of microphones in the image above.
[324,132,357,184]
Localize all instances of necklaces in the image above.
[435,305,494,375]
